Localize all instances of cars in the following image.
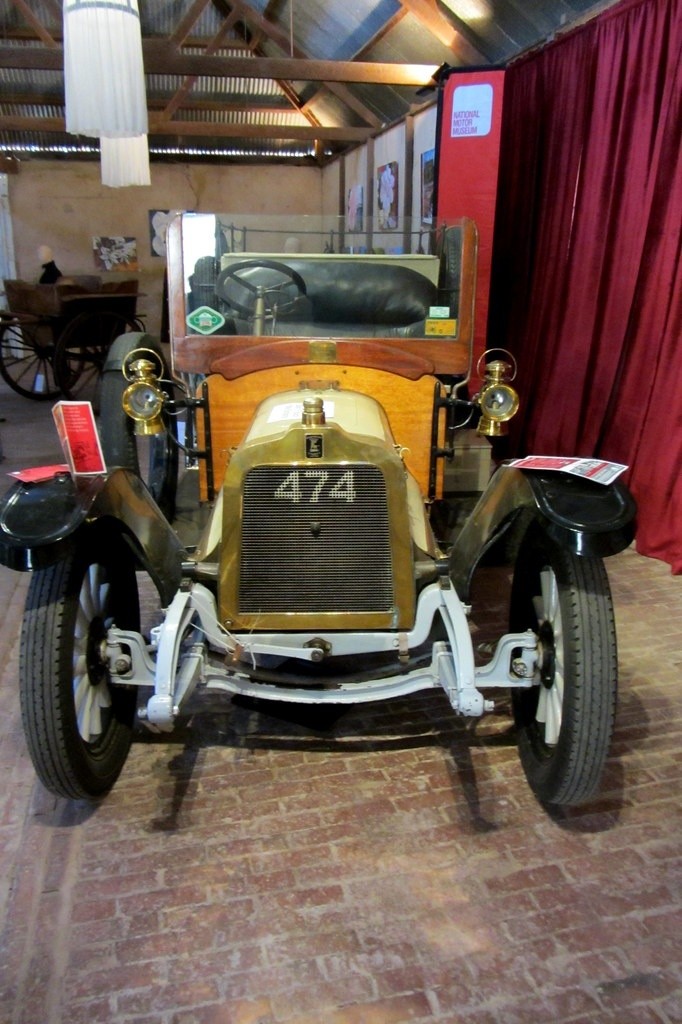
[1,212,639,821]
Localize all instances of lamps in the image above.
[62,0,151,189]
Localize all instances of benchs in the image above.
[215,258,439,336]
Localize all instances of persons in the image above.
[37,245,63,285]
